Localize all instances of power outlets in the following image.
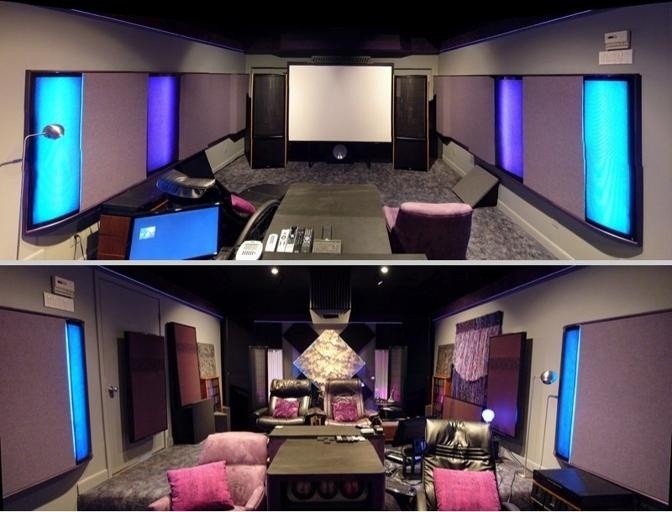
[70,228,81,248]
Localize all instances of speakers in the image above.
[392,74,429,173]
[245,71,287,168]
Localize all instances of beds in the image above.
[253,379,316,433]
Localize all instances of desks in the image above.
[263,184,392,258]
[266,438,385,511]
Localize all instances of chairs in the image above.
[383,202,473,260]
[157,169,288,248]
[147,430,271,511]
[412,418,521,511]
[316,379,383,434]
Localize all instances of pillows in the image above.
[272,399,300,419]
[433,468,501,511]
[332,402,358,422]
[166,460,235,511]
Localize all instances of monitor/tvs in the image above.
[391,417,426,447]
[124,201,223,260]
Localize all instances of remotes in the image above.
[300,226,314,255]
[323,435,366,444]
[264,233,278,253]
[322,223,332,240]
[276,228,289,253]
[284,226,298,253]
[293,228,304,254]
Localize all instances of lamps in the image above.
[17,124,64,259]
[516,370,557,479]
